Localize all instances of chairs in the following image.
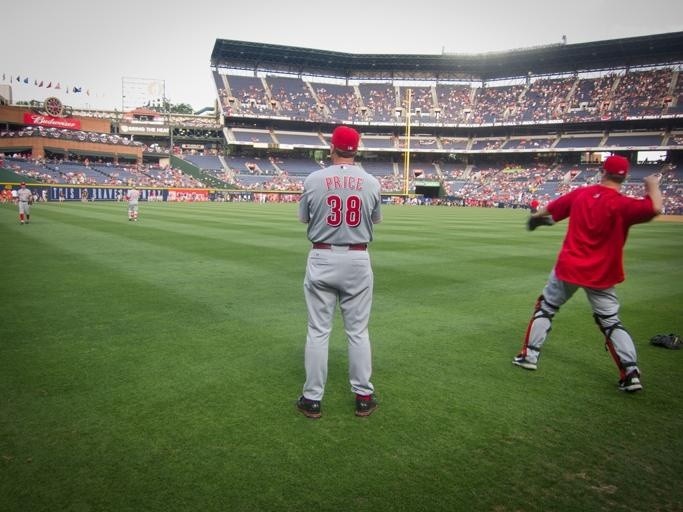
[222,72,682,212]
[0,138,222,188]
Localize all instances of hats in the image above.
[598,155,630,175]
[324,126,359,151]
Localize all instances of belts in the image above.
[312,243,366,250]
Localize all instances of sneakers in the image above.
[355,394,377,416]
[296,395,321,418]
[617,375,642,392]
[511,355,538,370]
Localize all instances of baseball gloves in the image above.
[529,217,552,229]
[651,334,682,349]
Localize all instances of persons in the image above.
[0,68,683,223]
[512,156,662,392]
[296,125,378,419]
